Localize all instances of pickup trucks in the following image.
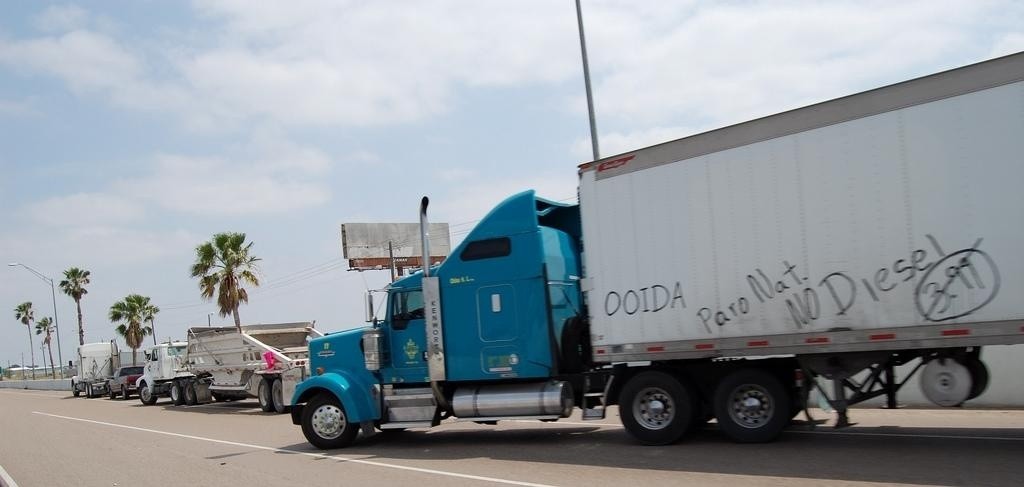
[107,366,144,400]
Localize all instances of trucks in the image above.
[71,342,145,398]
[291,50,1024,449]
[136,322,326,414]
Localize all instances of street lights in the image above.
[8,263,64,379]
